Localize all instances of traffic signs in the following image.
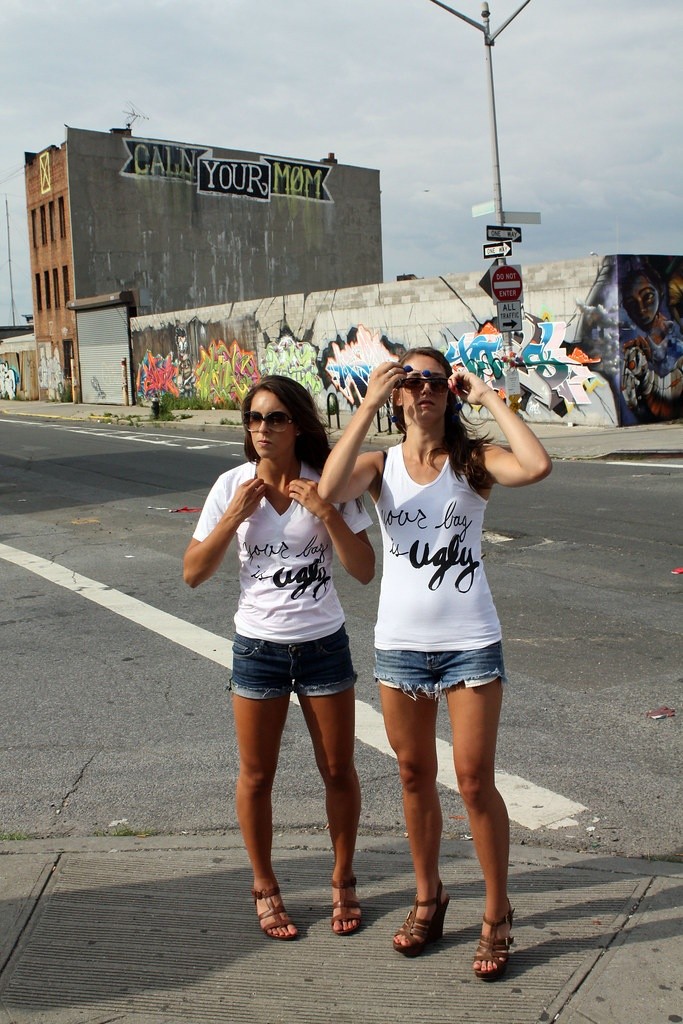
[482,225,523,332]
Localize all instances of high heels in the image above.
[392,879,450,956]
[472,896,517,981]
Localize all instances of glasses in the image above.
[243,410,294,433]
[395,377,449,393]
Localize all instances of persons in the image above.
[316,345,554,979]
[183,372,378,939]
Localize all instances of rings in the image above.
[389,369,395,375]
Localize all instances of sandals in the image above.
[330,878,362,935]
[250,886,298,941]
